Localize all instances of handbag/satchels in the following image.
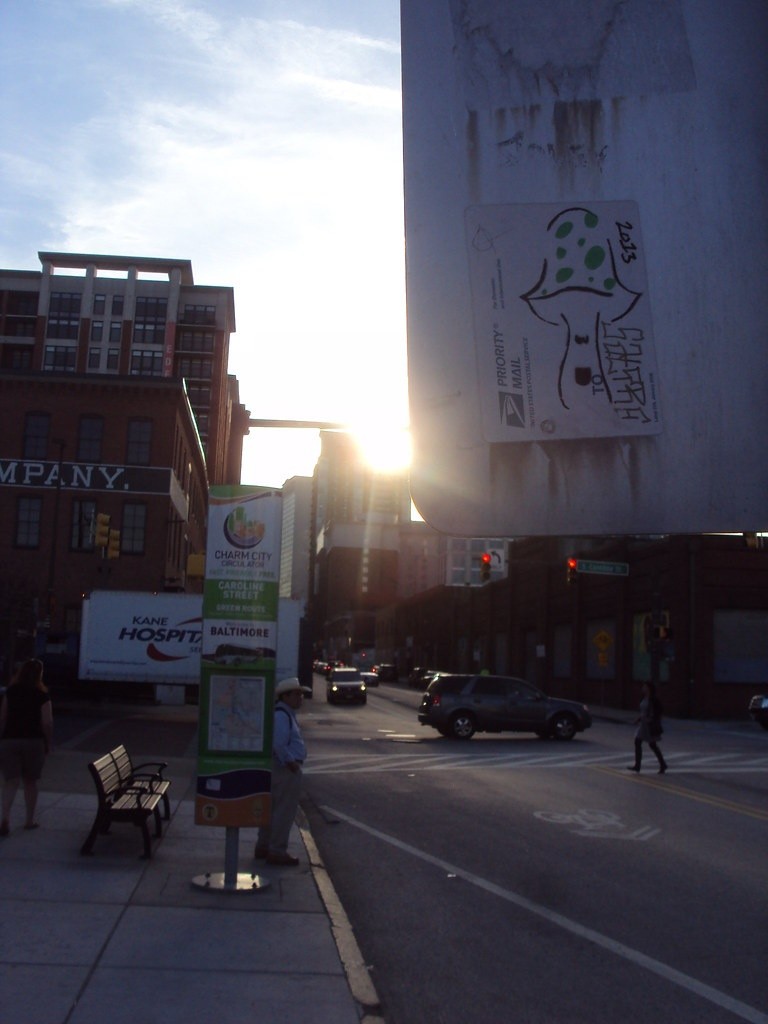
[648,720,664,736]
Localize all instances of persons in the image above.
[255,677,311,866]
[627,682,667,774]
[0,658,52,836]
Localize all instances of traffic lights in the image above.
[568,559,576,583]
[480,554,491,582]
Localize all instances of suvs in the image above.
[419,673,593,741]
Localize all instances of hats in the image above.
[275,678,311,695]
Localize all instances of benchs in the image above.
[81,740,171,860]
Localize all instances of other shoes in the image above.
[658,764,668,774]
[254,847,265,858]
[627,765,640,772]
[24,822,40,830]
[0,818,9,837]
[267,850,298,865]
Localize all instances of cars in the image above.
[326,668,367,705]
[408,667,446,689]
[311,657,399,686]
